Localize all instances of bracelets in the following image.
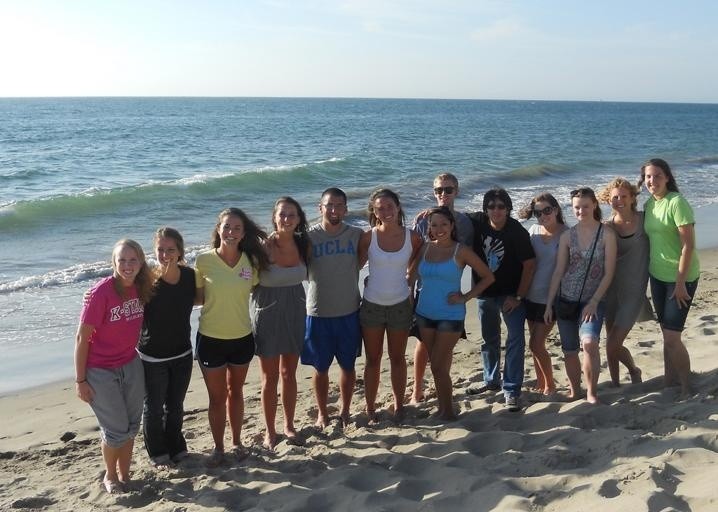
[461,292,473,302]
[74,377,86,384]
[592,293,600,306]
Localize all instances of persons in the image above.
[82,224,197,469]
[363,206,498,419]
[70,239,159,501]
[521,192,573,400]
[302,187,368,430]
[406,171,477,405]
[636,157,703,404]
[410,186,538,412]
[542,187,618,406]
[598,176,652,387]
[357,188,421,426]
[249,194,310,455]
[190,208,271,468]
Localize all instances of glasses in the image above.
[570,189,589,196]
[435,186,456,194]
[485,203,506,210]
[532,206,553,217]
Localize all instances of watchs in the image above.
[512,293,524,303]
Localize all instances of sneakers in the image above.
[465,383,501,395]
[505,397,520,411]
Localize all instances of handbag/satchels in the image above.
[558,300,581,321]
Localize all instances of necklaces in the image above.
[621,213,634,225]
